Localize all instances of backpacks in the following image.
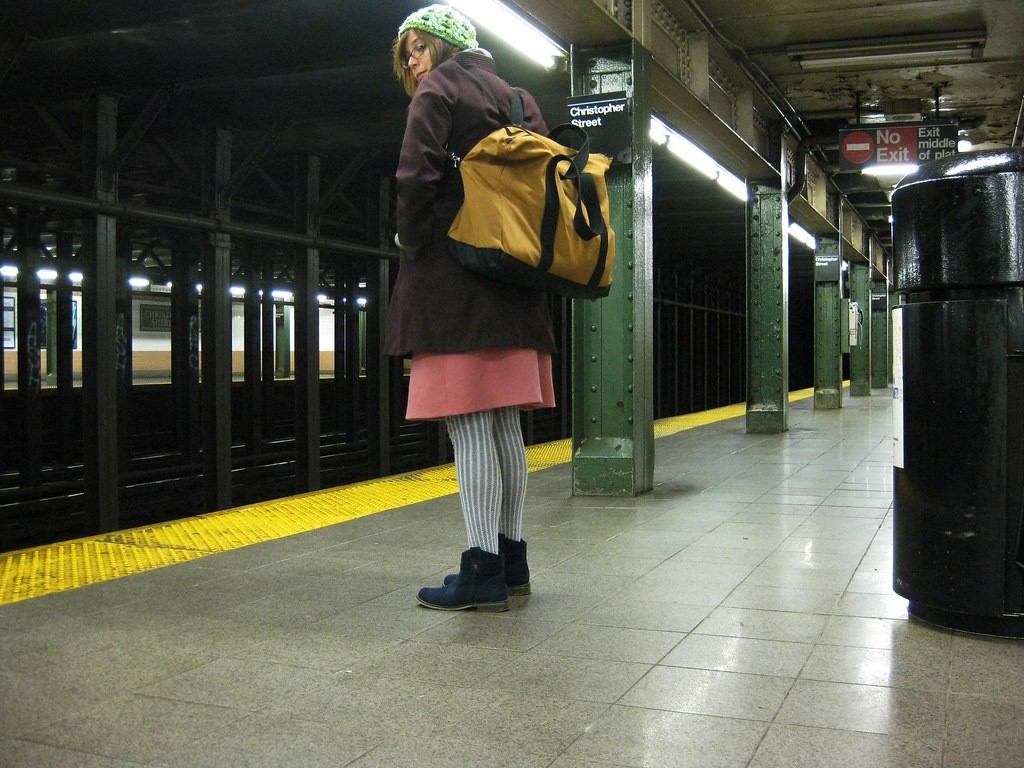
[443,87,615,302]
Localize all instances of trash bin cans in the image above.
[890,149,1024,639]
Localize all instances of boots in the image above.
[415,547,510,612]
[442,532,530,596]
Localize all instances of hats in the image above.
[398,4,479,50]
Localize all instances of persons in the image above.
[383,6,549,614]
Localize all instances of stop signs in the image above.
[842,132,874,163]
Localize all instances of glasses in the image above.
[400,43,426,69]
